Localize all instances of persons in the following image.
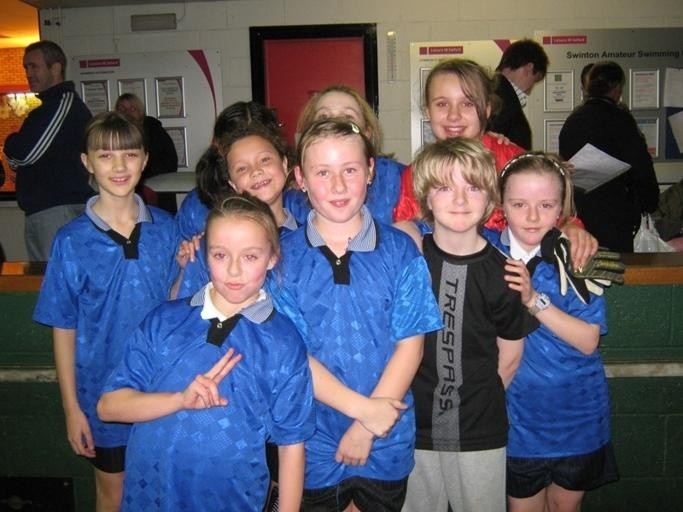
[1,39,94,260]
[386,152,619,509]
[178,86,406,297]
[115,92,176,215]
[97,194,315,511]
[32,112,205,509]
[394,60,599,271]
[262,116,445,511]
[398,140,537,511]
[487,42,550,150]
[559,61,659,252]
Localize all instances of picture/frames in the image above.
[541,69,576,114]
[418,65,431,111]
[80,78,111,118]
[634,116,660,160]
[418,117,437,151]
[541,118,569,157]
[626,66,663,111]
[116,77,149,117]
[151,74,189,120]
[163,125,189,170]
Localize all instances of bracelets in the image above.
[527,293,551,316]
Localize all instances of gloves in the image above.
[540,226,624,305]
